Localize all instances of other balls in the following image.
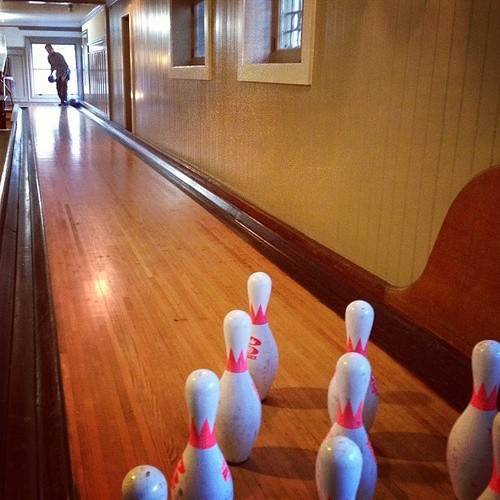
[69,98,75,105]
[47,76,54,83]
[75,102,80,108]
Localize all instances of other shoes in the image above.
[57,100,66,107]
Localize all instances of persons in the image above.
[44,43,70,106]
